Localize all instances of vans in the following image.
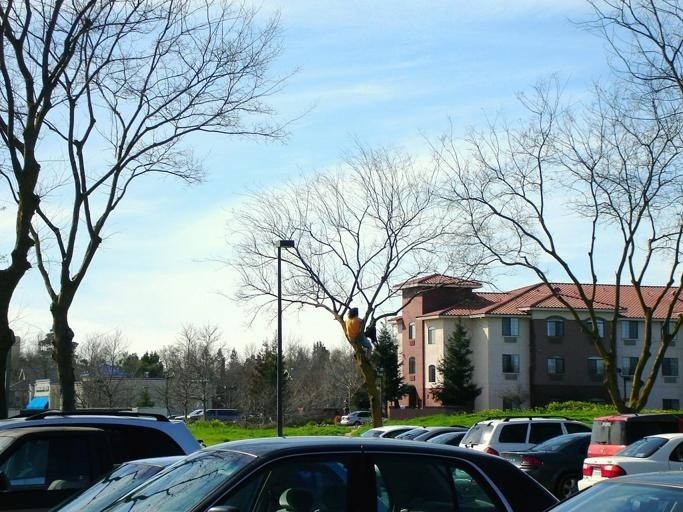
[167,408,243,426]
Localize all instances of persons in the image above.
[345,306,374,361]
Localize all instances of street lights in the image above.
[275,239,294,436]
